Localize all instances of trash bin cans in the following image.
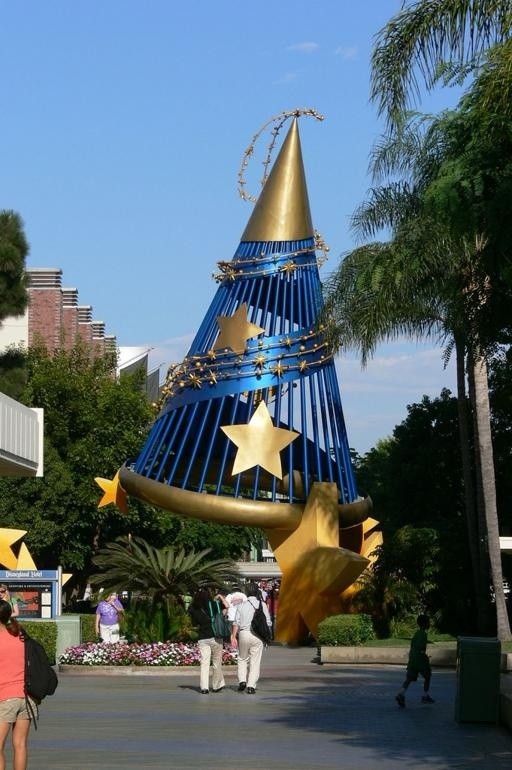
[457,637,502,724]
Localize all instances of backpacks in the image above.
[21,627,58,706]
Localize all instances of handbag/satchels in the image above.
[212,612,231,637]
[252,608,272,645]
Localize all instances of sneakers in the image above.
[238,682,255,694]
[396,693,406,709]
[421,695,436,705]
[202,686,223,694]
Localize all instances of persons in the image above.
[225,579,280,640]
[396,615,435,707]
[0,600,37,770]
[232,583,272,694]
[0,585,19,620]
[188,587,229,694]
[96,594,124,643]
